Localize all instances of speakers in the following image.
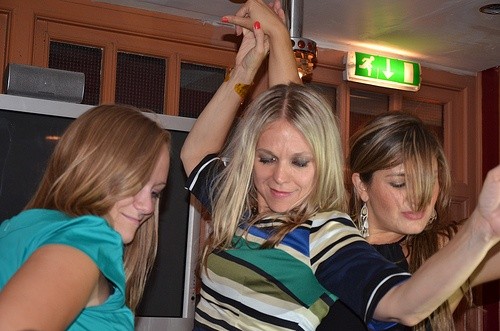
[2,62,85,103]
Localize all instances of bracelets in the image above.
[224,67,254,104]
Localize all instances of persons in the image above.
[180,0,500,331]
[0,104,170,331]
[220,0,500,331]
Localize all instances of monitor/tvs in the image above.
[0,94,203,331]
